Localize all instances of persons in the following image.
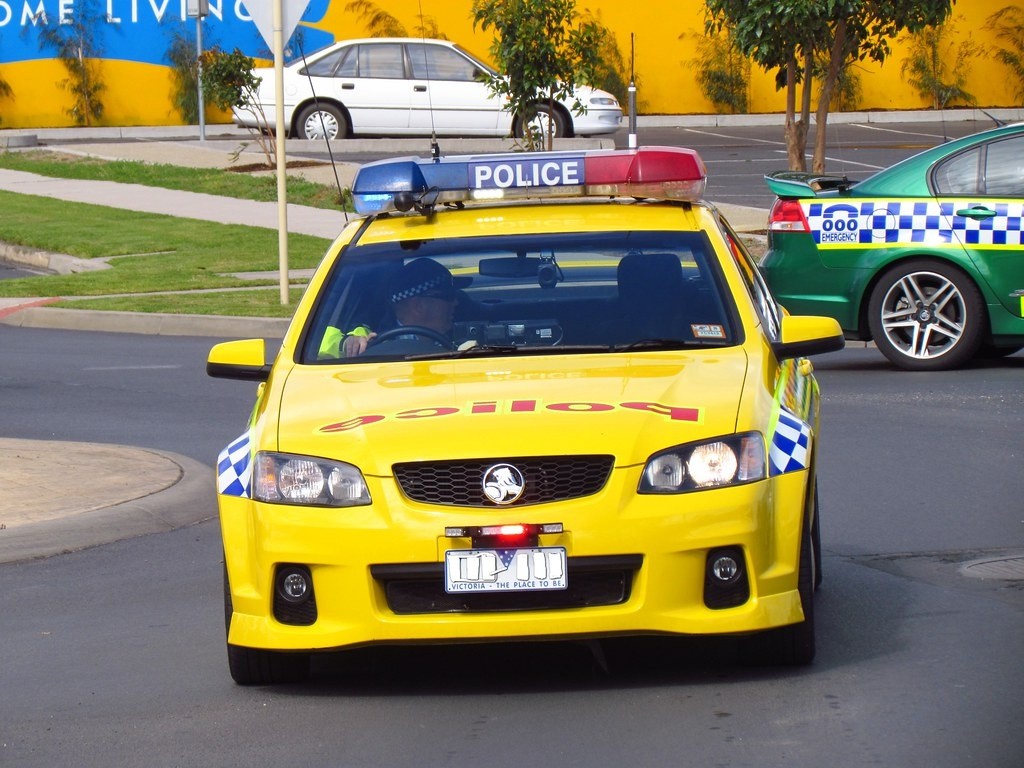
[316,261,472,359]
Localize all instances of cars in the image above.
[201,140,849,690]
[231,35,624,140]
[750,118,1024,372]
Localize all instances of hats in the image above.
[390,257,474,304]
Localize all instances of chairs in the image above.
[563,253,718,345]
